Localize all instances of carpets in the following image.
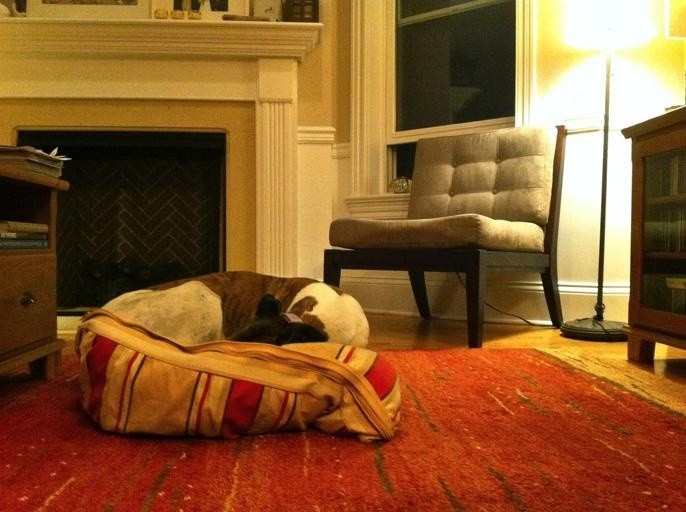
[0,343,686,512]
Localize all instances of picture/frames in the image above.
[168,0,250,21]
[26,0,154,20]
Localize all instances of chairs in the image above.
[324,125,565,349]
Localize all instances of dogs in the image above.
[97,269,371,346]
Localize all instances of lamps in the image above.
[560,1,659,343]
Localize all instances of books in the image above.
[0,145,73,180]
[0,220,50,250]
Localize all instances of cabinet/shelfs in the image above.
[620,105,686,363]
[0,164,71,382]
